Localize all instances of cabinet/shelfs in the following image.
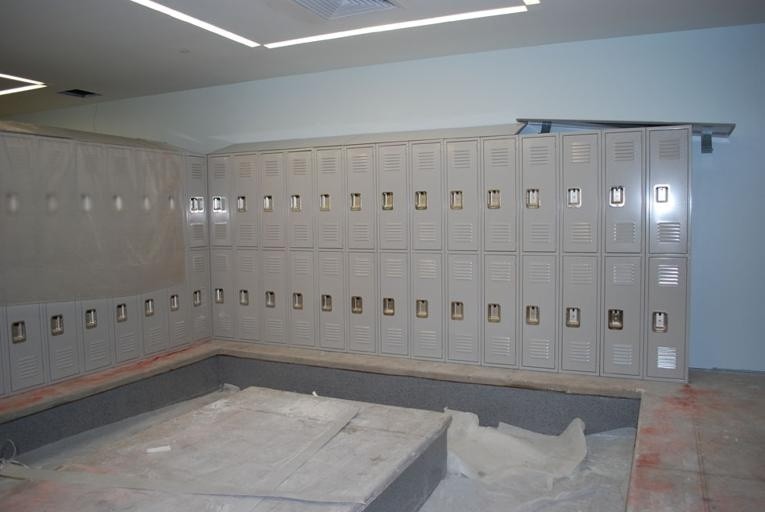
[208,115,736,385]
[0,118,207,399]
